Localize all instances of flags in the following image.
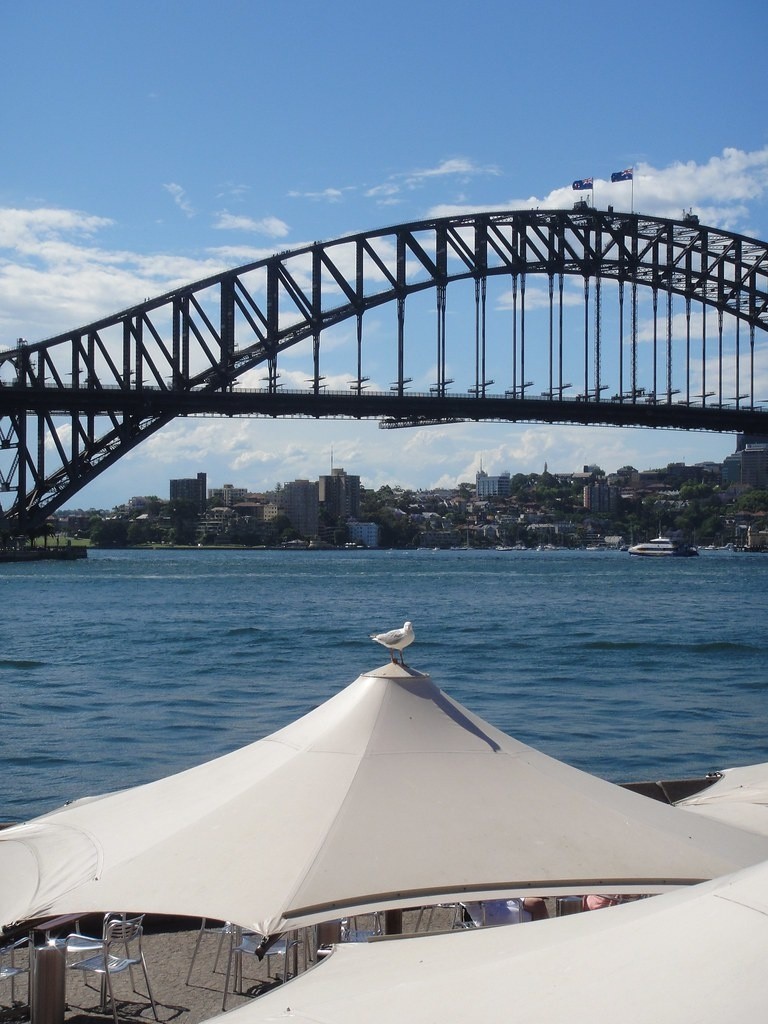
[611,167,632,183]
[572,178,593,190]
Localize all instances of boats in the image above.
[719,543,734,550]
[586,543,607,551]
[628,531,697,556]
[308,539,335,549]
[415,523,473,550]
[537,545,545,552]
[497,546,513,551]
[705,544,718,550]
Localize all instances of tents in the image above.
[0,660,768,1024]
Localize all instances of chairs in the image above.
[0,937,33,1020]
[65,912,158,1024]
[185,896,583,1010]
[67,912,135,1007]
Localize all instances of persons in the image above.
[458,898,549,927]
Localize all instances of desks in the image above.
[29,913,100,985]
